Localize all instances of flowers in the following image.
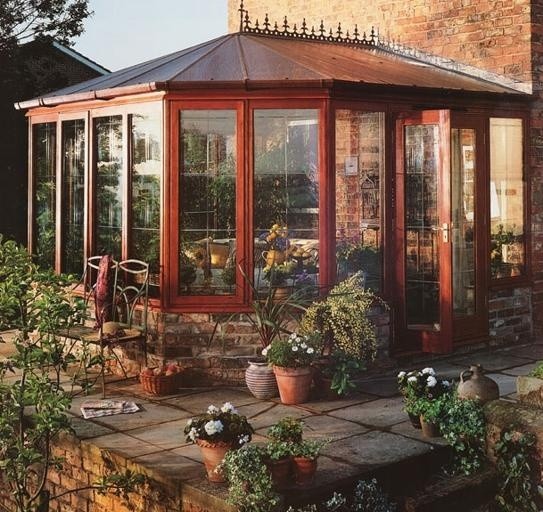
[184,402,254,448]
[259,224,290,252]
[267,334,318,369]
[398,368,456,416]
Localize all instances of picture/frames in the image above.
[343,155,359,176]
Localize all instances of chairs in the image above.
[38,257,114,395]
[80,260,154,401]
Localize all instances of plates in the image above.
[287,254,313,271]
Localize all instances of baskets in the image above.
[140,362,184,395]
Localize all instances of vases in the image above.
[407,412,421,430]
[273,365,312,404]
[262,250,286,266]
[196,439,234,482]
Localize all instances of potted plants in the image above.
[297,267,393,398]
[262,416,305,474]
[408,393,451,434]
[262,263,296,302]
[213,264,358,400]
[291,440,327,483]
